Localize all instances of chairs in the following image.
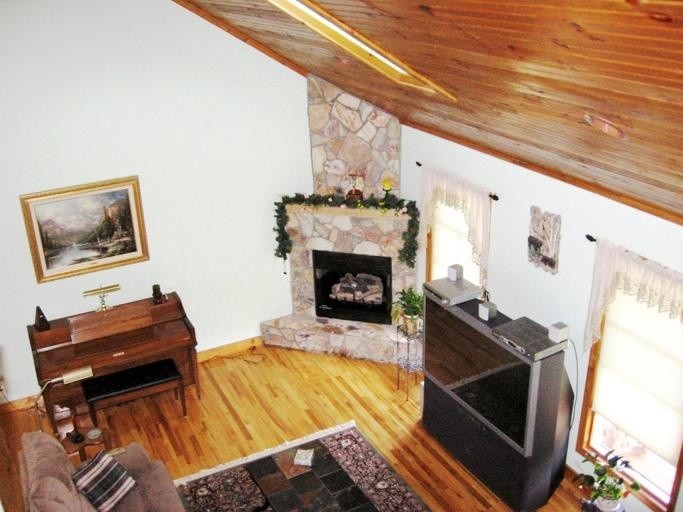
[16,443,185,512]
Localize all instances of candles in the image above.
[383,176,392,190]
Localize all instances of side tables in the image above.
[60,423,111,464]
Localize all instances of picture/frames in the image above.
[19,174,150,283]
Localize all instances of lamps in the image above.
[83,285,122,315]
[34,366,94,426]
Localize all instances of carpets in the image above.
[173,421,431,512]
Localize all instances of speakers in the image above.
[478,302,497,321]
[549,322,570,344]
[447,264,462,281]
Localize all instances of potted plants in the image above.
[572,449,642,512]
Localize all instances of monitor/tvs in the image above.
[422,288,542,458]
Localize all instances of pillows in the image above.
[22,431,82,512]
[72,449,137,512]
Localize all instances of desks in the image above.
[26,291,201,439]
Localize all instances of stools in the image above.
[397,327,424,401]
[82,359,187,430]
[387,289,425,335]
